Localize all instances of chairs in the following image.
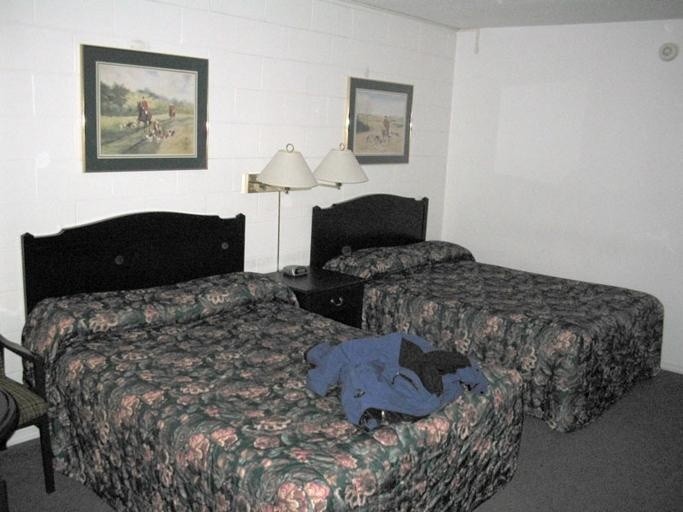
[1,332,54,493]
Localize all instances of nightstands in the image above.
[268,263,363,327]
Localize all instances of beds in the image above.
[310,194,663,432]
[21,211,524,512]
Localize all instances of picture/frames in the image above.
[80,44,209,172]
[348,76,413,164]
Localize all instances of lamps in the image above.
[244,142,369,192]
